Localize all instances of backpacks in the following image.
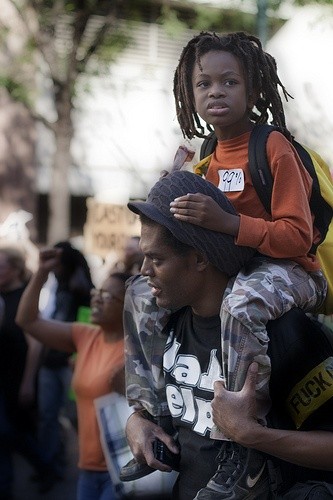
[200,124,333,255]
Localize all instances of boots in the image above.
[118,409,176,482]
[193,443,277,500]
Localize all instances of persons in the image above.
[0,242,146,500]
[126,169,333,499]
[118,30,333,500]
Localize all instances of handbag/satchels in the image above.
[95,392,178,496]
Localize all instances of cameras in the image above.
[155,437,180,473]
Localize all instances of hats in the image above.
[126,171,243,277]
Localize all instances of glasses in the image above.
[90,288,122,305]
